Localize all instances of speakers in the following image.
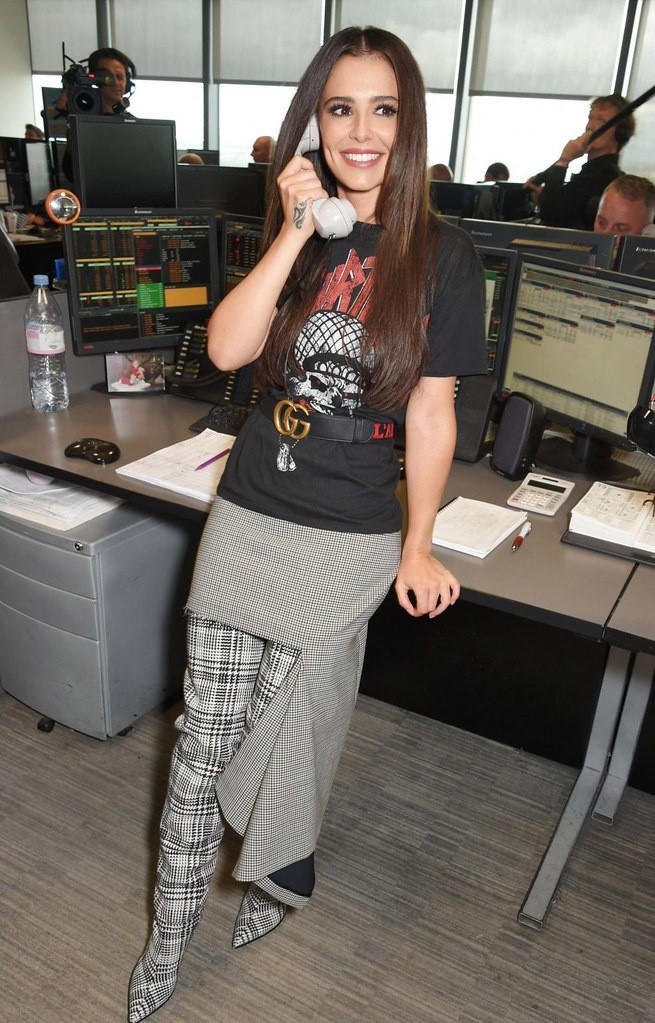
[491,392,547,480]
[454,370,498,463]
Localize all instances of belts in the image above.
[257,392,394,444]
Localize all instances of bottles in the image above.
[23,273,70,415]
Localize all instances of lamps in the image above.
[36,189,80,225]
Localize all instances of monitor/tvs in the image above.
[0,86,655,480]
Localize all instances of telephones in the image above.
[292,112,358,239]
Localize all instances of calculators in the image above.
[507,471,575,516]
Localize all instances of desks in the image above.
[0,385,655,935]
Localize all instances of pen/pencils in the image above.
[511,521,532,552]
[195,448,231,471]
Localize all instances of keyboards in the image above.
[188,404,256,437]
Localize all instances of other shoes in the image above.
[127,923,198,1023]
[233,880,287,948]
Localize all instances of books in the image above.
[431,495,528,559]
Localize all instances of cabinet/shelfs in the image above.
[0,499,210,741]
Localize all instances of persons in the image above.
[124,26,489,1023]
[430,163,454,182]
[56,47,136,182]
[178,154,205,164]
[119,360,145,386]
[250,135,274,163]
[593,174,655,250]
[26,124,44,140]
[476,162,509,183]
[524,93,638,231]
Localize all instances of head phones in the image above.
[89,48,137,93]
